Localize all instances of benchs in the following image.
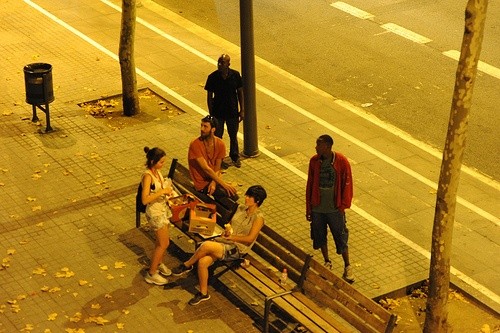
[134,157,398,333]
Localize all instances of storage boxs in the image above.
[188,202,217,236]
[166,193,199,223]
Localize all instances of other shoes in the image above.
[221,161,228,169]
[229,152,241,168]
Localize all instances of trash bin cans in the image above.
[22,62,55,105]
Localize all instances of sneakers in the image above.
[144,272,168,285]
[189,291,210,305]
[343,264,354,282]
[171,263,193,276]
[324,260,333,270]
[157,263,172,276]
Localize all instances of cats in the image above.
[221,222,234,238]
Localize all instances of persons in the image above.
[204,54,244,169]
[305,134,356,283]
[171,185,267,306]
[188,115,240,202]
[141,146,173,286]
